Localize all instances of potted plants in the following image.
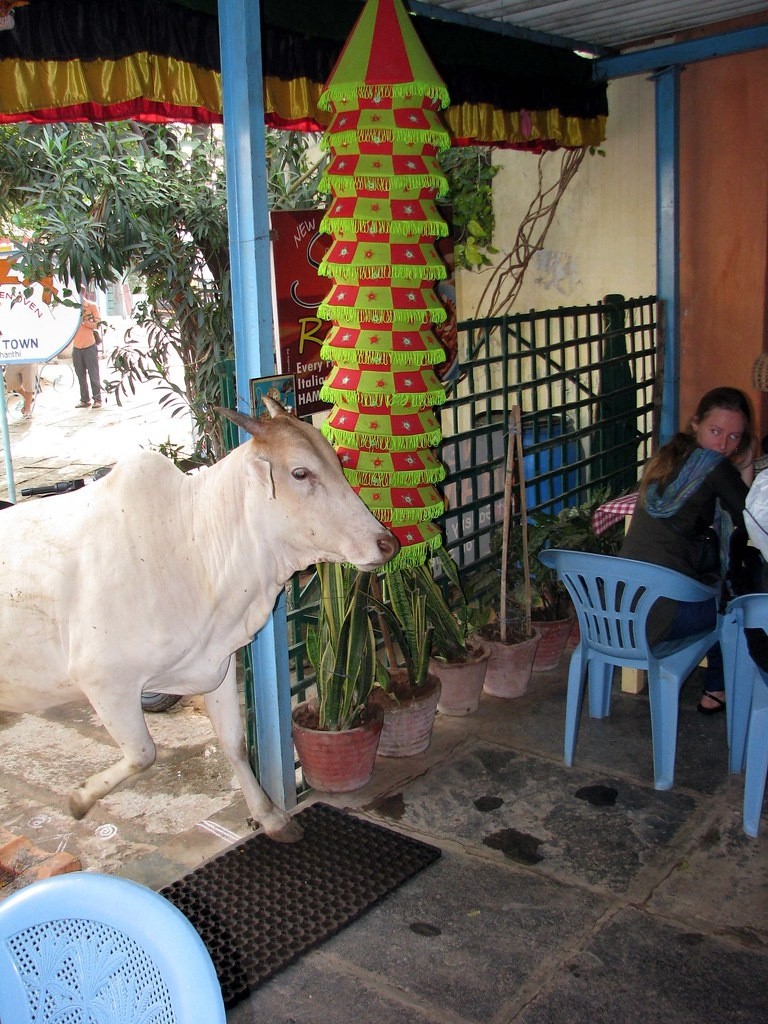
[292,482,635,792]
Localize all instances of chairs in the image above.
[724,593,768,838]
[0,871,226,1024]
[537,548,734,792]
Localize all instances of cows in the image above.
[0,394,401,844]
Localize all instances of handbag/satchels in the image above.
[93,330,101,345]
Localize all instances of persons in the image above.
[6,364,38,418]
[72,284,101,409]
[613,387,768,715]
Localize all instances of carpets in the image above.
[156,801,440,1007]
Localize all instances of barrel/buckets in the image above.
[472,407,585,581]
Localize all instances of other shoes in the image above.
[21,407,31,419]
[92,401,102,408]
[74,402,89,408]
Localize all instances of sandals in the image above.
[696,690,726,714]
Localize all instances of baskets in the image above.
[752,354,768,392]
